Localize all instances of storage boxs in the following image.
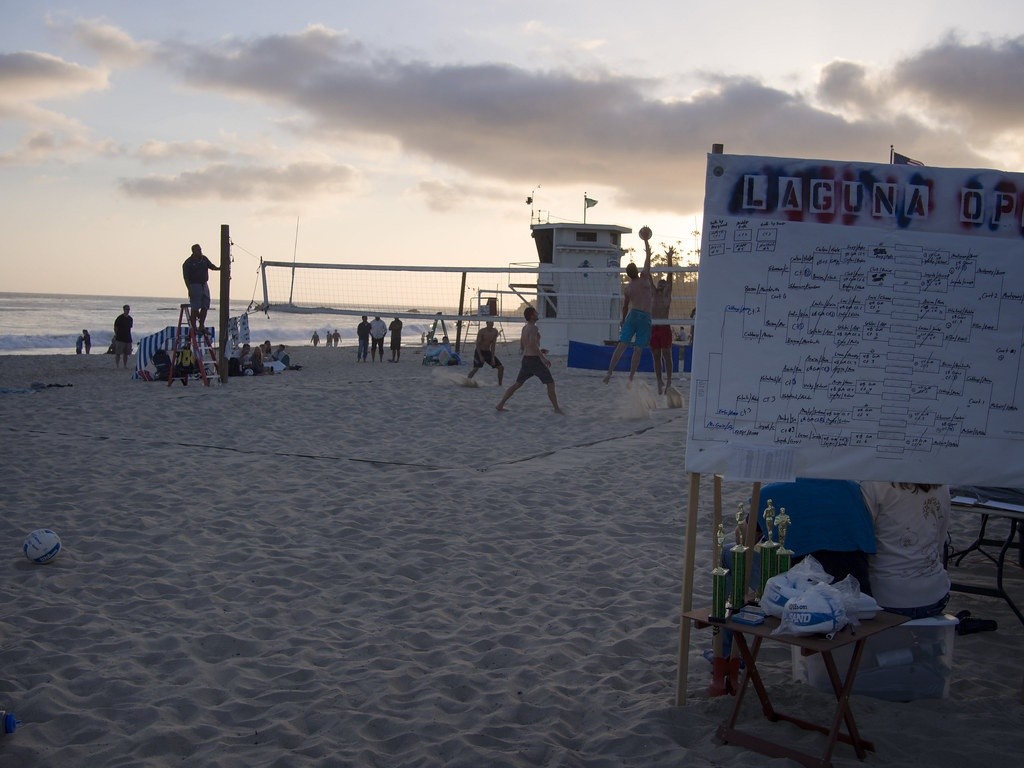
[791,613,958,701]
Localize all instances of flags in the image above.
[894,152,924,166]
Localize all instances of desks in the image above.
[943,496,1024,626]
[681,602,919,768]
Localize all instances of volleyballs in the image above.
[639,227,652,240]
[23,528,62,564]
[245,369,253,376]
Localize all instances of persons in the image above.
[496,307,564,414]
[858,480,952,619]
[356,316,387,362]
[468,321,504,386]
[603,239,653,383]
[703,477,878,672]
[426,336,461,366]
[231,340,290,375]
[676,307,696,344]
[108,305,133,368]
[182,244,220,335]
[311,332,320,347]
[647,248,674,395]
[388,318,402,363]
[326,330,342,348]
[76,330,91,355]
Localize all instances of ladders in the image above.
[462,321,480,353]
[167,304,225,387]
[428,312,448,339]
